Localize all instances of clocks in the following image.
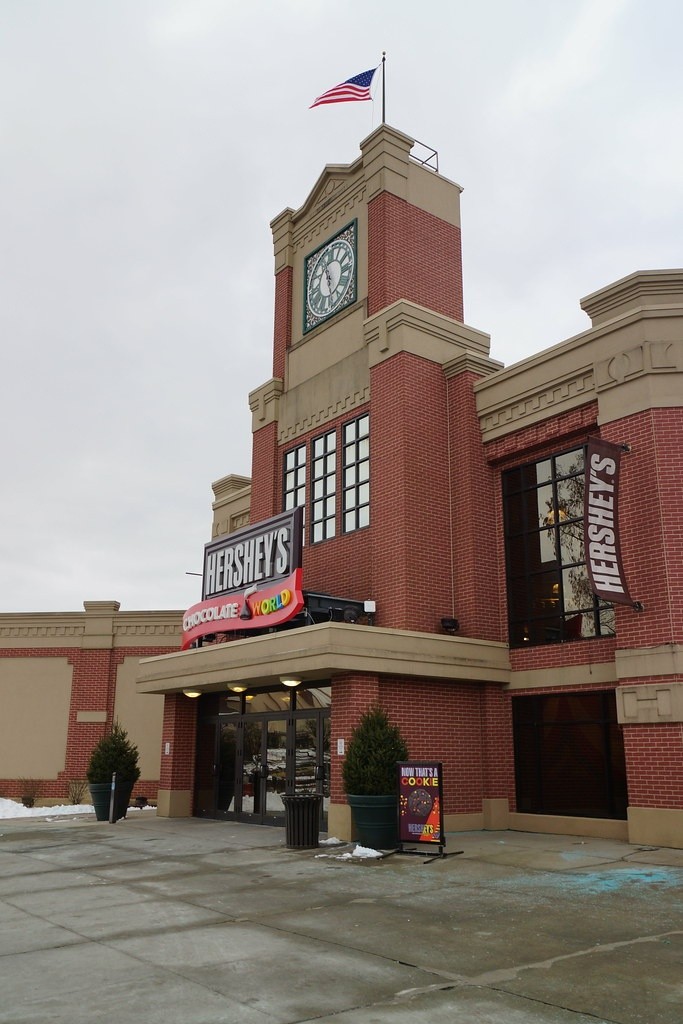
[302,217,357,335]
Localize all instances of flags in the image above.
[308,63,380,109]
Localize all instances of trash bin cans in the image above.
[280,793,325,850]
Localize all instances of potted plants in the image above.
[342,706,409,850]
[136,798,148,808]
[16,776,45,808]
[86,716,141,821]
